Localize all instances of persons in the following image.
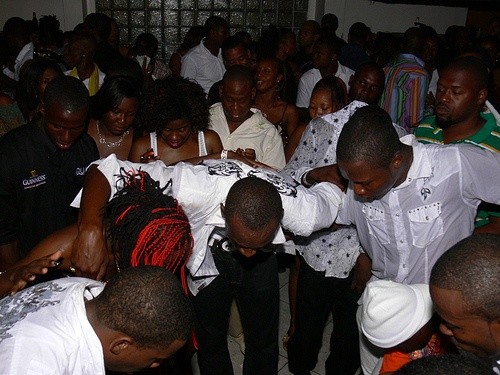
[0,13,500,375]
